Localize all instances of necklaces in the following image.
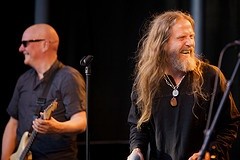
[161,70,187,106]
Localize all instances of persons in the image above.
[127,11,240,160]
[0,23,88,160]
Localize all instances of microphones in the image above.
[129,153,140,160]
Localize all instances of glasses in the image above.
[21,39,45,47]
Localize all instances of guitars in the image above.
[9,101,58,160]
